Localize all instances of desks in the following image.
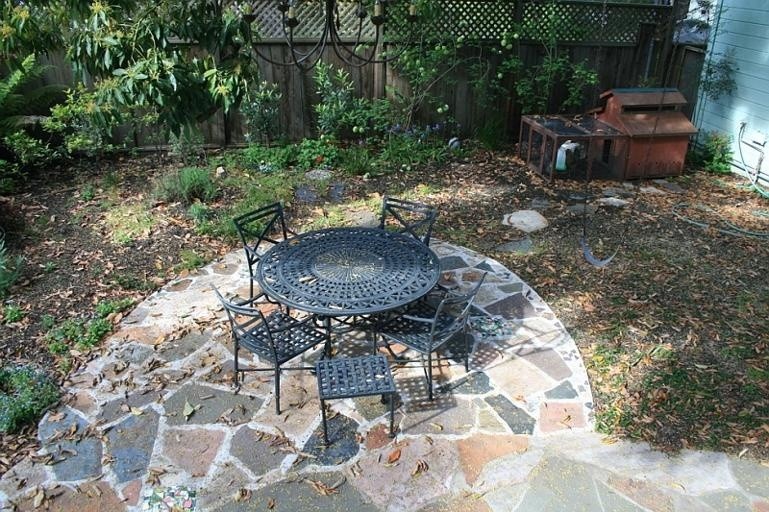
[255,226,443,375]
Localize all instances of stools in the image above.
[315,355,396,446]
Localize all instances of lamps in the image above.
[243,0,421,73]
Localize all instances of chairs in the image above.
[375,271,488,401]
[233,202,298,316]
[215,289,330,416]
[379,196,440,302]
